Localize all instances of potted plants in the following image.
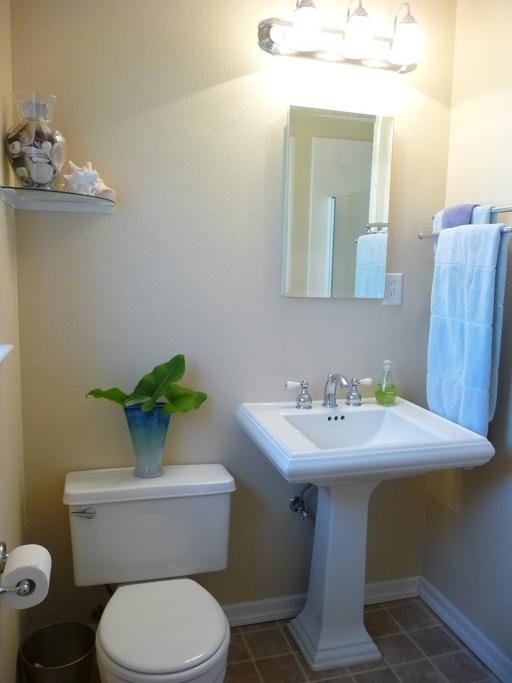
[85,353,209,478]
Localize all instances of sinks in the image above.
[233,395,496,486]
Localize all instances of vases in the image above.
[2,87,68,190]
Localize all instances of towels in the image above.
[425,222,510,438]
[429,202,495,257]
[441,203,480,228]
[354,231,387,298]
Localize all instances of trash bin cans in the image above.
[18,619,99,683]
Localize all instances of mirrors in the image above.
[280,104,394,301]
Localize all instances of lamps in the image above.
[257,0,426,75]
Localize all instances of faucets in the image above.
[320,374,349,407]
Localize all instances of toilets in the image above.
[62,463,236,682]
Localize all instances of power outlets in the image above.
[385,274,403,305]
